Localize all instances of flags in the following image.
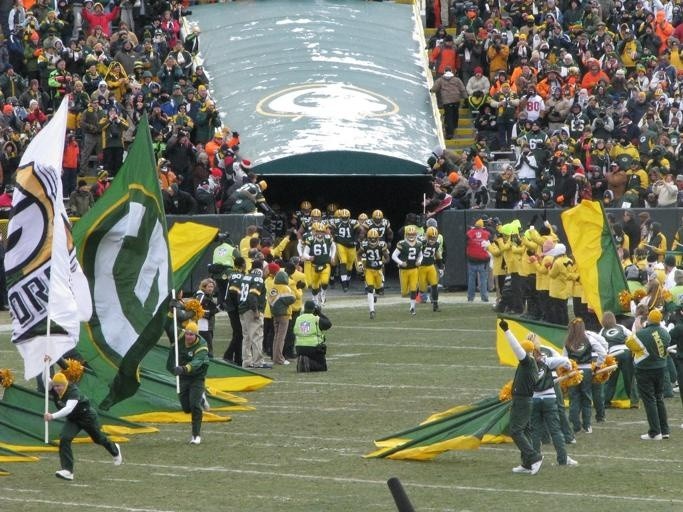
[358,314,645,461]
[0,321,283,479]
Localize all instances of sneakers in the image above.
[190,435,201,445]
[512,465,531,474]
[56,470,73,480]
[641,433,663,441]
[567,456,577,465]
[531,455,545,475]
[199,392,209,410]
[112,443,122,466]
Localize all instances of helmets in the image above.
[301,201,350,233]
[367,229,379,238]
[427,226,438,238]
[372,210,384,219]
[358,213,369,220]
[405,225,417,235]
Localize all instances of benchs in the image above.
[424,38,486,167]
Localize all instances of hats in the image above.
[185,322,198,334]
[521,340,534,351]
[649,310,662,323]
[53,373,67,386]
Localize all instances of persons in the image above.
[37,353,123,482]
[491,311,546,475]
[597,310,640,409]
[561,317,612,437]
[668,313,682,405]
[624,306,671,440]
[157,298,209,447]
[526,345,580,469]
[1,0,682,377]
[584,330,609,425]
[526,332,577,446]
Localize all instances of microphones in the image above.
[387,477,414,512]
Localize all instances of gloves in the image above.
[170,299,176,313]
[174,366,183,376]
[499,318,509,332]
